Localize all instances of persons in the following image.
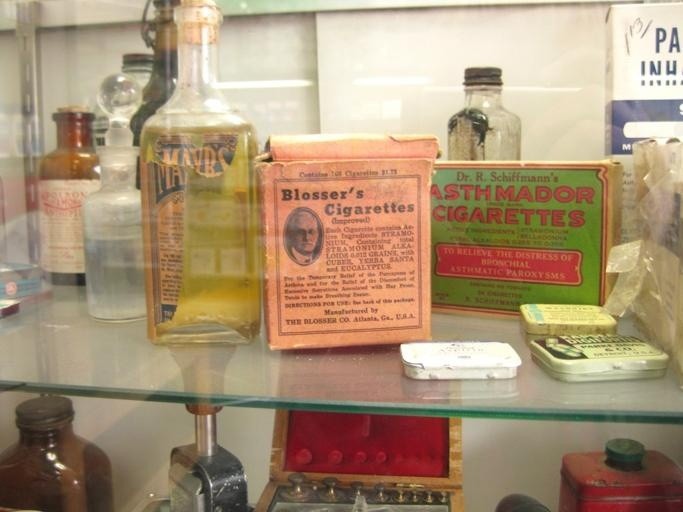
[289,211,319,264]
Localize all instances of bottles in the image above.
[129,0,183,190]
[445,67,521,161]
[137,1,265,348]
[37,100,95,288]
[83,75,152,324]
[2,394,115,510]
